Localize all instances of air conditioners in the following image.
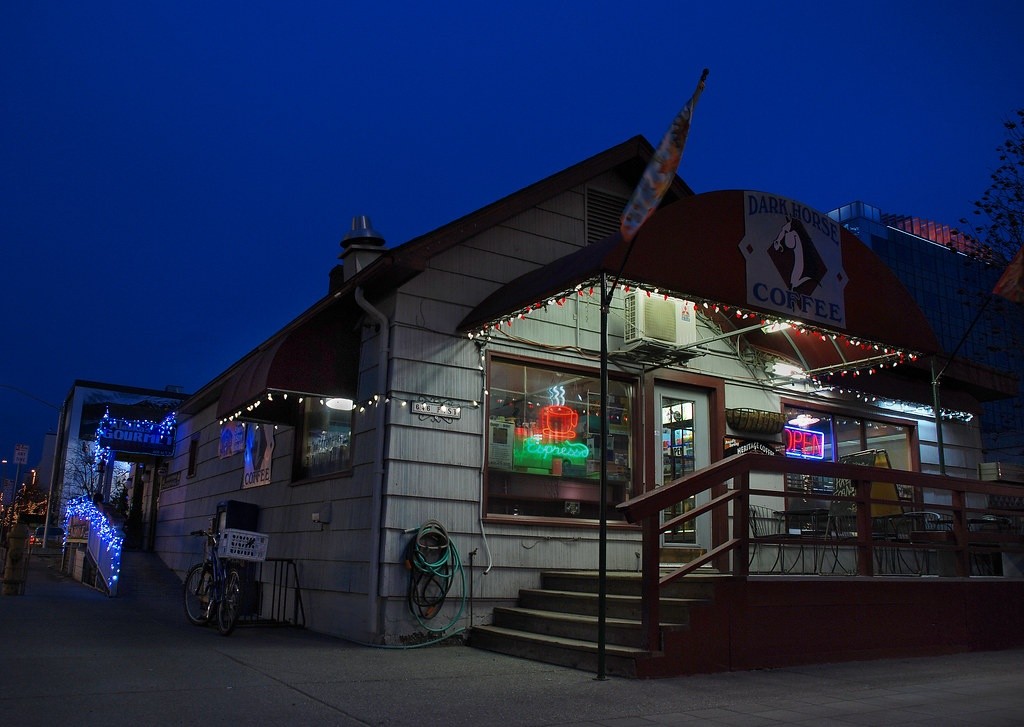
[623,289,697,349]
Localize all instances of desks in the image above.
[874,513,926,576]
[929,519,1003,576]
[775,509,840,574]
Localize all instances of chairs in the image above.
[747,504,1024,575]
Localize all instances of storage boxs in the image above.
[977,461,1024,482]
[218,528,270,562]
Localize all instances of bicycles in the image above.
[182,529,247,636]
